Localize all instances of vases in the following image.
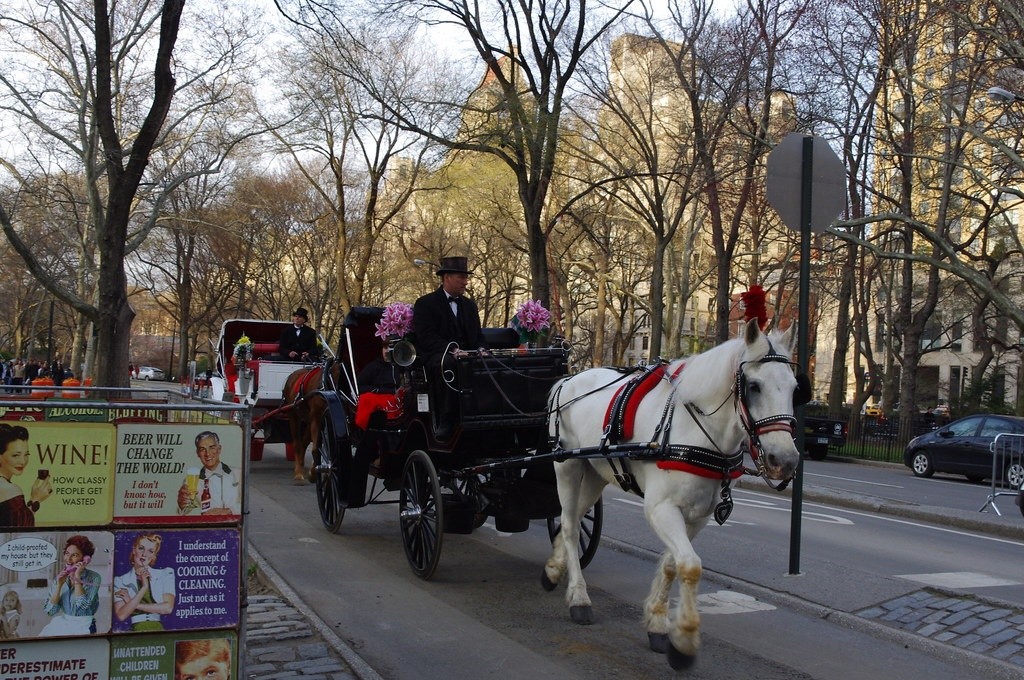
[237,358,245,371]
[526,342,537,351]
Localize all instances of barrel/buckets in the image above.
[441,485,480,535]
[250,438,265,461]
[286,441,298,461]
[495,480,529,533]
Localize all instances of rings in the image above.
[49,489,52,494]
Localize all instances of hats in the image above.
[292,308,308,322]
[436,257,474,277]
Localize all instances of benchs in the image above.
[397,325,570,413]
[225,343,280,384]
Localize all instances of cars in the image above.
[131,364,166,382]
[195,371,217,387]
[903,413,1024,490]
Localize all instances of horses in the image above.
[540,316,803,673]
[280,357,339,485]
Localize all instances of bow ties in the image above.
[447,296,461,305]
[295,327,304,331]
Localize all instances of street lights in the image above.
[413,258,476,302]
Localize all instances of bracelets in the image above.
[27,501,40,512]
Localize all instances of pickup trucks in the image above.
[797,414,848,461]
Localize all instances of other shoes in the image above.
[434,422,451,441]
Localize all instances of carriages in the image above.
[207,316,336,486]
[314,306,804,672]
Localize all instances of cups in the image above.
[186,465,200,499]
[38,468,49,480]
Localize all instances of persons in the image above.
[358,336,399,428]
[113,531,176,632]
[175,638,232,680]
[135,364,139,379]
[0,591,24,639]
[0,356,71,408]
[177,431,240,515]
[128,363,133,376]
[924,407,936,432]
[0,423,53,527]
[37,535,101,636]
[414,257,488,440]
[279,307,318,365]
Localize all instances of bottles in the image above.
[201,478,211,515]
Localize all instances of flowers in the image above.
[233,334,253,362]
[510,299,550,342]
[375,303,413,339]
